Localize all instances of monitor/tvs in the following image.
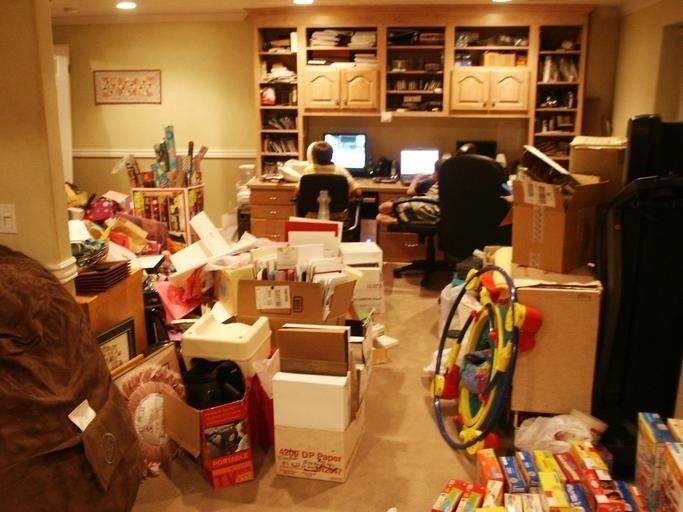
[323,132,368,175]
[456,139,497,160]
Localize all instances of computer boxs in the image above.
[359,216,378,242]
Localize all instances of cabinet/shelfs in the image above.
[524,1,597,160]
[248,5,305,180]
[384,5,447,119]
[298,5,385,117]
[483,243,605,429]
[447,5,536,117]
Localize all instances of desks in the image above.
[243,168,508,264]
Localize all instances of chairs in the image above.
[284,173,363,231]
[387,151,503,288]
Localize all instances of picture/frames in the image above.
[92,69,162,105]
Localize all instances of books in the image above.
[309,29,442,112]
[263,116,298,153]
[264,67,297,83]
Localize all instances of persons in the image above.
[295,141,362,229]
[407,158,447,197]
[375,181,442,226]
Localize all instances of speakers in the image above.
[495,153,507,169]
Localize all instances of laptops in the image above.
[399,147,440,185]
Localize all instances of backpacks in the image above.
[0,244,143,512]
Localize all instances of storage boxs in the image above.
[130,182,203,255]
[566,132,629,191]
[501,163,611,274]
[59,213,385,491]
